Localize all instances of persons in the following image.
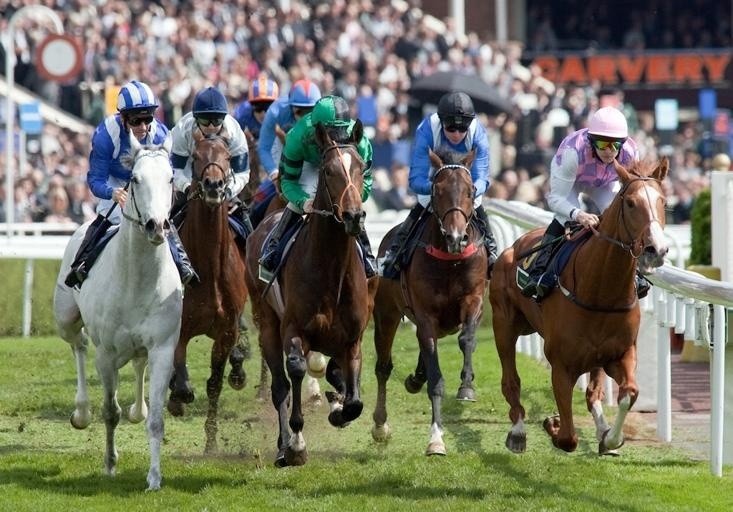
[1,0,733,300]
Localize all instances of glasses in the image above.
[123,115,156,127]
[195,116,225,128]
[443,121,469,134]
[588,137,625,152]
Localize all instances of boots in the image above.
[166,219,202,287]
[62,216,108,288]
[520,232,559,299]
[356,229,380,280]
[381,210,420,277]
[258,207,302,272]
[476,210,498,268]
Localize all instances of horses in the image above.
[487,154,671,457]
[255,120,327,415]
[50,128,186,495]
[239,126,267,205]
[367,145,490,460]
[177,121,248,459]
[241,117,379,468]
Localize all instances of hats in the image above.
[115,79,159,113]
[287,79,322,108]
[191,85,231,117]
[586,106,629,140]
[247,75,280,105]
[310,95,352,130]
[437,91,476,120]
